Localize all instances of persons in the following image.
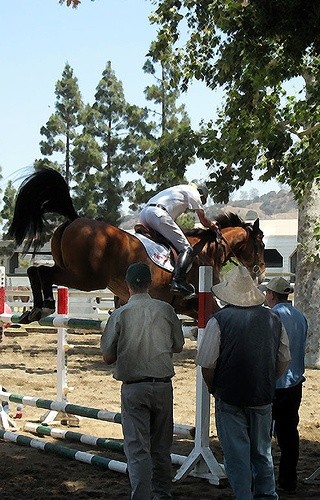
[137,184,219,300]
[193,267,308,500]
[100,263,185,500]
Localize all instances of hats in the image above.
[257,277,294,294]
[125,263,151,285]
[210,265,266,307]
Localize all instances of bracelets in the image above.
[211,223,215,228]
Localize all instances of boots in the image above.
[169,246,193,296]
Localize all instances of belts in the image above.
[123,377,171,384]
[149,204,166,210]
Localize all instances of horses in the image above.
[6,162,268,326]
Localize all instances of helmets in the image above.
[191,179,208,205]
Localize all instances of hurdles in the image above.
[0,262,228,490]
